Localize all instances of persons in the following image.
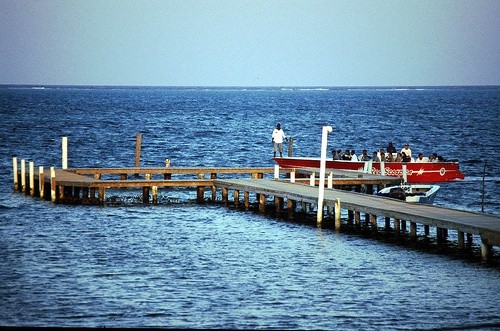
[350,150,358,162]
[379,149,395,162]
[272,124,288,158]
[395,152,411,163]
[342,150,352,160]
[429,153,445,162]
[371,151,380,162]
[400,144,412,158]
[332,149,342,160]
[387,141,396,153]
[415,153,429,162]
[357,150,371,161]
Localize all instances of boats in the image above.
[379,184,442,206]
[275,154,465,180]
